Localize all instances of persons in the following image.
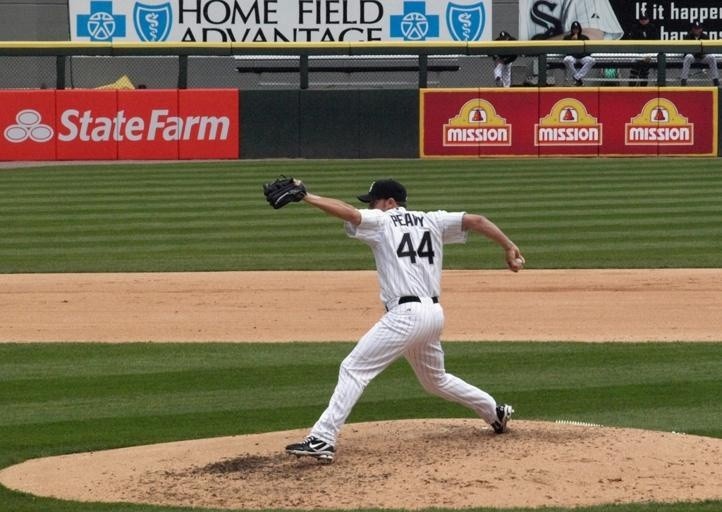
[557,21,598,84]
[677,20,721,86]
[263,173,526,462]
[626,16,661,85]
[490,31,520,86]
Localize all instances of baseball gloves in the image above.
[262,176,305,209]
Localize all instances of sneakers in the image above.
[681,79,686,86]
[491,403,513,434]
[571,76,584,86]
[495,76,500,85]
[713,79,720,86]
[286,436,336,462]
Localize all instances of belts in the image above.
[398,296,439,305]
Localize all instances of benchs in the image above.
[540,60,721,86]
[232,64,459,88]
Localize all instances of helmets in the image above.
[500,31,507,38]
[571,21,581,29]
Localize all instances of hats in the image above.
[357,178,407,203]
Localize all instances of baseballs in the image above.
[516,258,523,265]
